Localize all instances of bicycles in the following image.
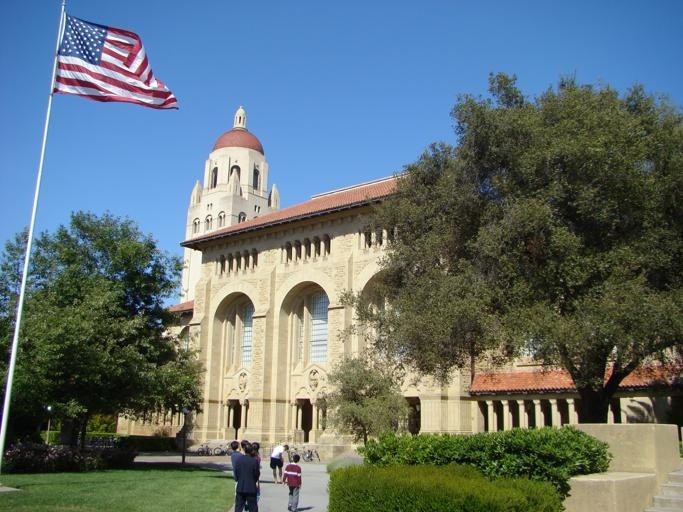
[197,441,212,456]
[288,445,321,463]
[214,441,234,456]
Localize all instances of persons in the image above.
[229,441,243,477]
[240,437,250,457]
[269,443,289,483]
[250,441,261,504]
[238,372,247,391]
[308,369,318,391]
[282,454,302,512]
[235,443,258,512]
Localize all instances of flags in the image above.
[52,12,181,111]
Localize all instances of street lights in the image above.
[45,405,54,444]
[182,407,190,464]
